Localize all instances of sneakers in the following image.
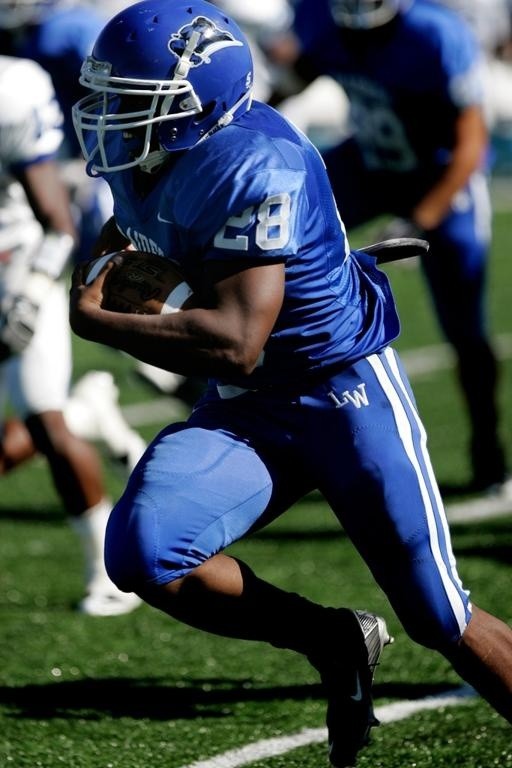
[306,606,394,767]
[71,368,147,470]
[78,551,145,618]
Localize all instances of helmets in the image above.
[71,0,255,201]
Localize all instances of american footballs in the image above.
[82,252,200,312]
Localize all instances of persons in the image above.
[1,1,512,615]
[69,0,512,768]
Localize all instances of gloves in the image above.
[0,296,42,357]
